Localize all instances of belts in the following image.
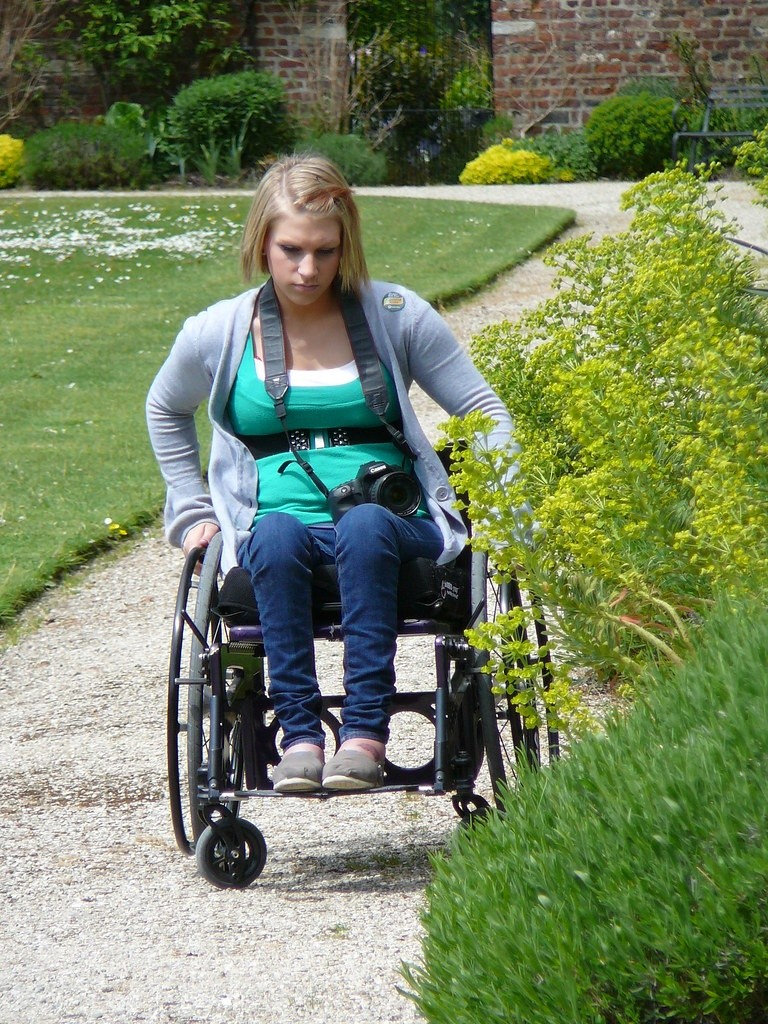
[240,425,403,462]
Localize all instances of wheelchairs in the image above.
[166,439,564,890]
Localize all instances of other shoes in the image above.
[271,744,325,793]
[322,735,387,790]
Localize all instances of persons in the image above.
[146,156,534,793]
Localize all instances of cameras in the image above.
[328,460,422,526]
